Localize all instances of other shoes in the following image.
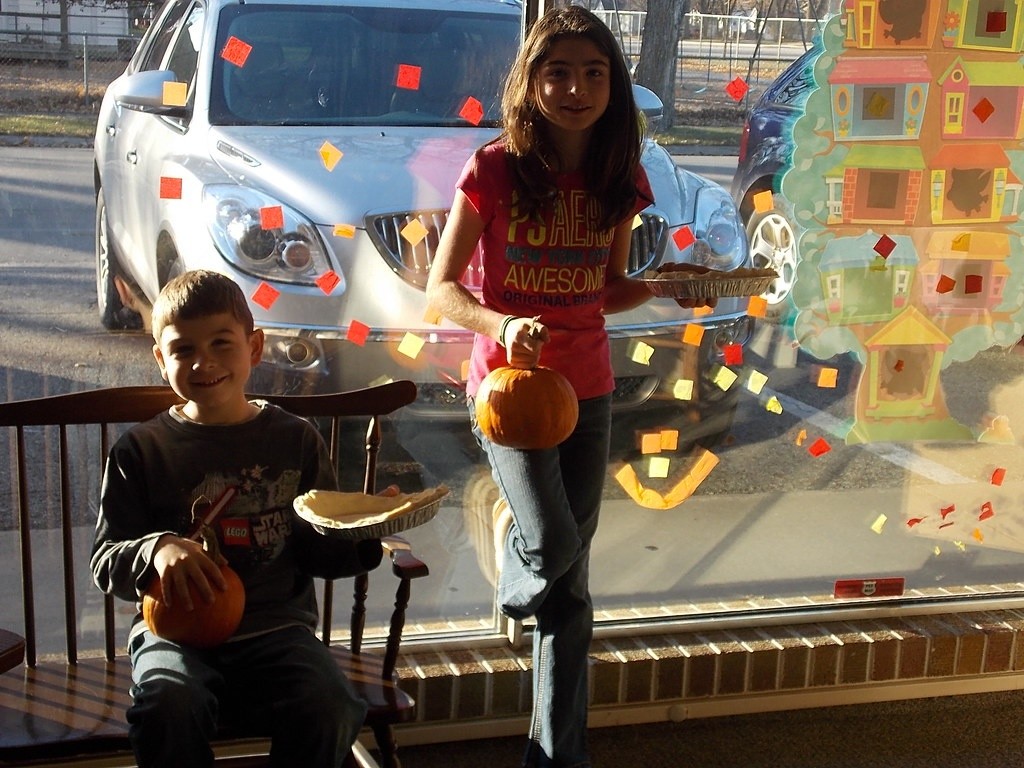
[491,499,514,567]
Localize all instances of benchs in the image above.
[0,380,429,768]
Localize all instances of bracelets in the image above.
[502,316,520,346]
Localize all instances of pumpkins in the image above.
[142,564,245,648]
[476,364,579,449]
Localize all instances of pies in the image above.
[643,266,779,279]
[292,484,449,528]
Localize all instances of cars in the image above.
[88,0,756,447]
[729,42,828,327]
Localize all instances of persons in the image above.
[423,4,718,768]
[88,270,400,768]
